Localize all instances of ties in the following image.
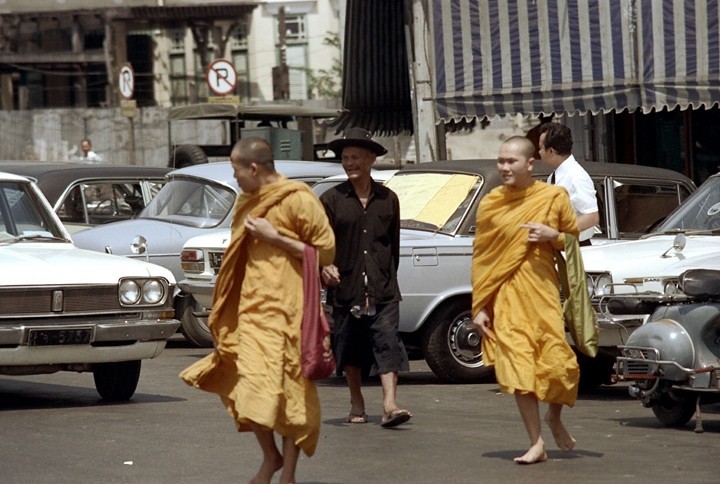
[549,172,556,185]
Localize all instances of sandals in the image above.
[347,398,370,424]
[379,408,412,429]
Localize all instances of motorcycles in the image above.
[600,265,720,435]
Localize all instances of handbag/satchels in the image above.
[300,245,336,381]
[555,233,602,361]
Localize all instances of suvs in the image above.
[163,102,347,166]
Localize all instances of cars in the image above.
[0,159,181,233]
[383,156,699,385]
[0,170,184,402]
[70,160,348,348]
[180,168,400,317]
[570,168,720,395]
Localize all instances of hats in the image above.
[327,127,388,158]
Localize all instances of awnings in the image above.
[421,0,719,125]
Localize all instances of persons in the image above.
[80,139,103,163]
[531,123,601,249]
[176,134,336,484]
[470,135,580,465]
[313,128,412,427]
